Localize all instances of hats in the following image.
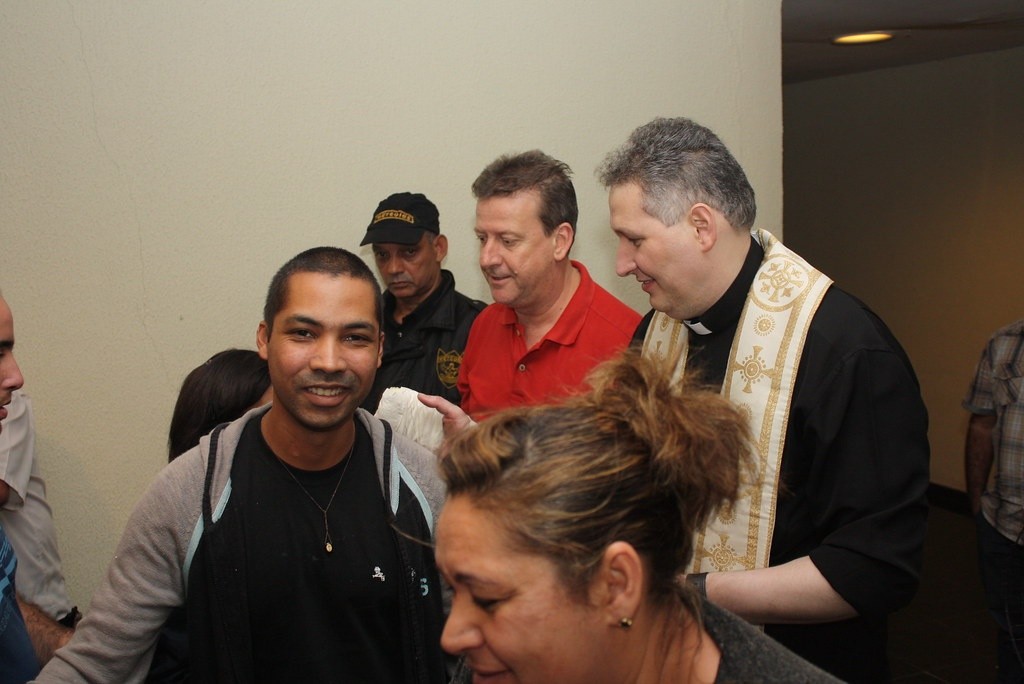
[360,192,440,245]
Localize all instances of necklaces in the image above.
[266,423,355,551]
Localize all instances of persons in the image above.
[361,192,489,416]
[962,318,1024,684]
[168,349,278,463]
[0,393,83,626]
[458,148,646,422]
[417,114,932,684]
[32,246,449,684]
[0,299,75,684]
[435,349,848,684]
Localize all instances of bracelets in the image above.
[685,573,706,599]
